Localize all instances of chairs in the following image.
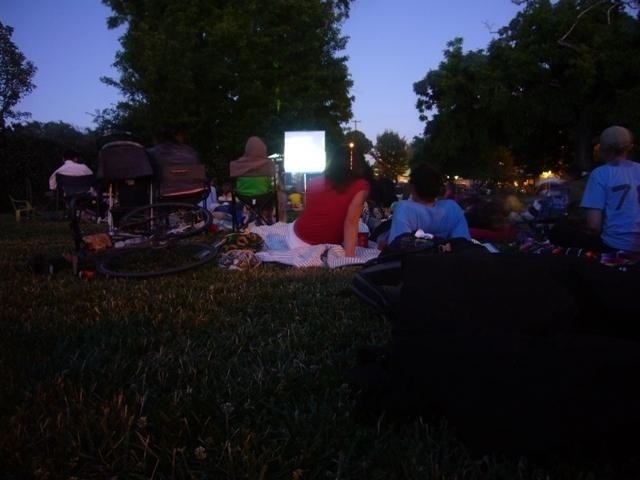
[55,141,279,237]
[9,194,38,224]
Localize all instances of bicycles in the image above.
[44,200,218,280]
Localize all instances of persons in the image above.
[550,124,640,253]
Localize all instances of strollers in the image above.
[528,187,570,233]
